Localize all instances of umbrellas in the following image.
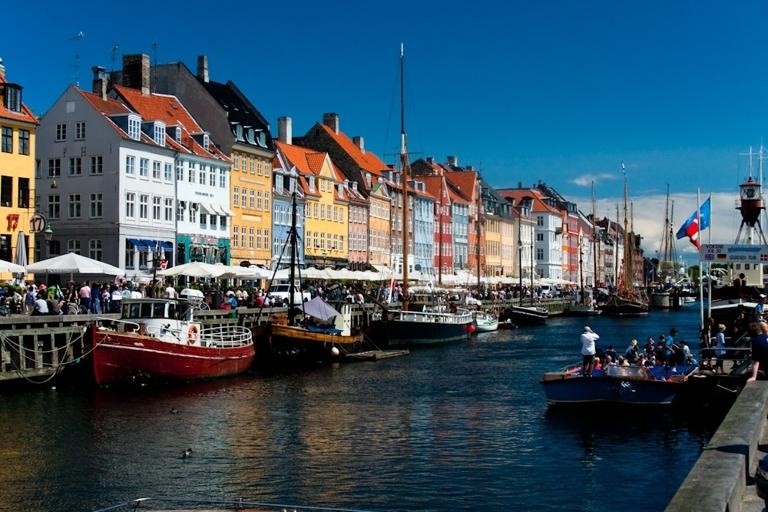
[0,230,126,281]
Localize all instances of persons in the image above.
[580,294,768,378]
[1,279,698,317]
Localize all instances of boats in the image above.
[253,192,365,369]
[541,357,696,403]
[93,288,256,386]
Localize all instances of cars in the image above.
[267,282,313,309]
[543,283,555,297]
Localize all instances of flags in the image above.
[676,196,710,250]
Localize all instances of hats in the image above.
[39,284,46,290]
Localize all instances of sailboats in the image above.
[500,210,548,324]
[368,44,498,346]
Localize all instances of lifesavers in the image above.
[188,325,198,344]
